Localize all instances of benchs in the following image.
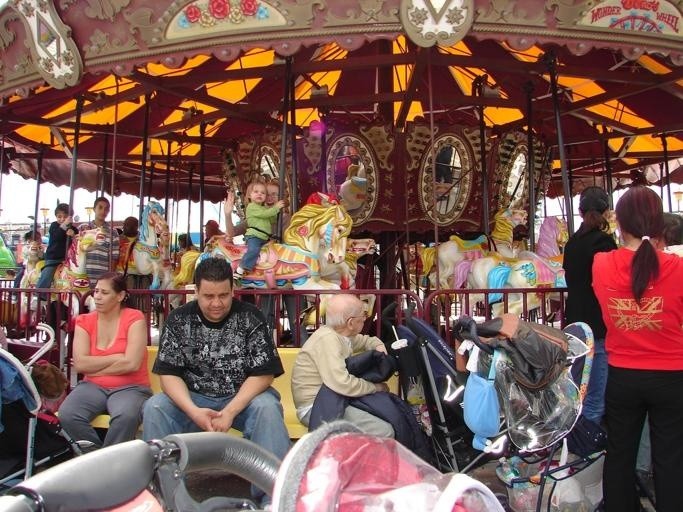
[45,342,402,444]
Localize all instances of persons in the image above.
[560,183,682,512]
[0,178,394,507]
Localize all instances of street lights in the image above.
[84,206,94,230]
[39,207,49,236]
[672,186,682,212]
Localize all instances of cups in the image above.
[391,338,408,351]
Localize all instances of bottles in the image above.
[500,457,520,483]
[420,405,433,437]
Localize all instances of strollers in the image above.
[0,420,508,511]
[445,312,652,512]
[0,323,82,495]
[375,295,516,478]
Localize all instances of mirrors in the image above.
[259,155,284,214]
[333,144,368,214]
[431,143,463,216]
[504,151,527,210]
